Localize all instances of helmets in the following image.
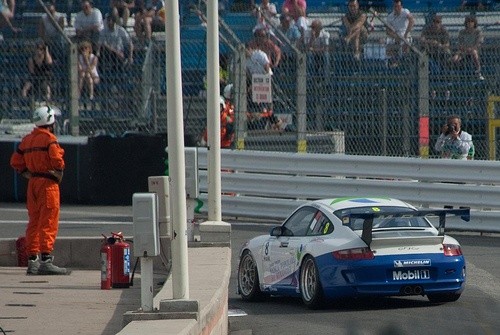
[219,97,226,110]
[33,105,55,126]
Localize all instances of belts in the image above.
[29,172,60,183]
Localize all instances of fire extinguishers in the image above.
[108,233,133,289]
[98,232,111,290]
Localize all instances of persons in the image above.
[245,39,273,80]
[75,43,101,99]
[0,0,491,74]
[22,41,56,102]
[199,53,233,111]
[10,106,72,274]
[434,114,473,160]
[203,98,235,150]
[447,15,486,80]
[223,83,274,125]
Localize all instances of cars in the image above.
[236,195,471,308]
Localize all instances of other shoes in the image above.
[27,255,40,275]
[38,256,72,276]
